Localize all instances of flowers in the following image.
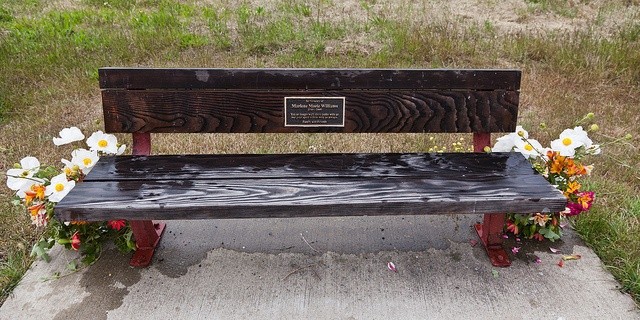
[482,125,601,242]
[5,128,136,264]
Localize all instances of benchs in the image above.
[54,66,567,267]
